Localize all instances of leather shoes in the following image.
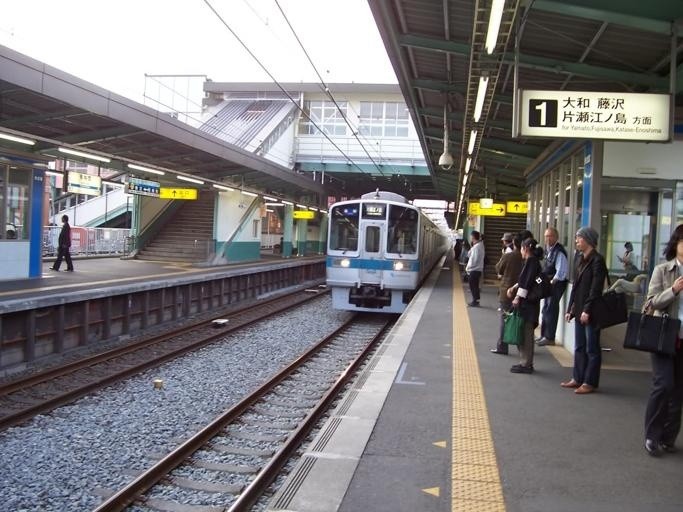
[660,436,677,456]
[536,337,555,346]
[560,377,580,387]
[64,269,74,272]
[533,336,544,343]
[49,267,59,272]
[575,383,597,393]
[644,437,662,457]
[490,349,509,355]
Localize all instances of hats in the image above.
[576,227,599,248]
[501,232,513,241]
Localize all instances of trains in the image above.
[325,189,449,315]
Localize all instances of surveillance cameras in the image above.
[438,152,454,171]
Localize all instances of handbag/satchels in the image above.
[503,305,525,346]
[527,273,555,302]
[592,291,628,330]
[622,311,681,356]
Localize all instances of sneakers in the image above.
[512,365,534,370]
[467,300,480,306]
[510,367,534,374]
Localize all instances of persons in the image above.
[454,231,485,308]
[491,228,647,394]
[50,215,73,271]
[641,224,683,457]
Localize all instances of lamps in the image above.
[467,127,478,155]
[473,71,490,123]
[484,0,506,55]
